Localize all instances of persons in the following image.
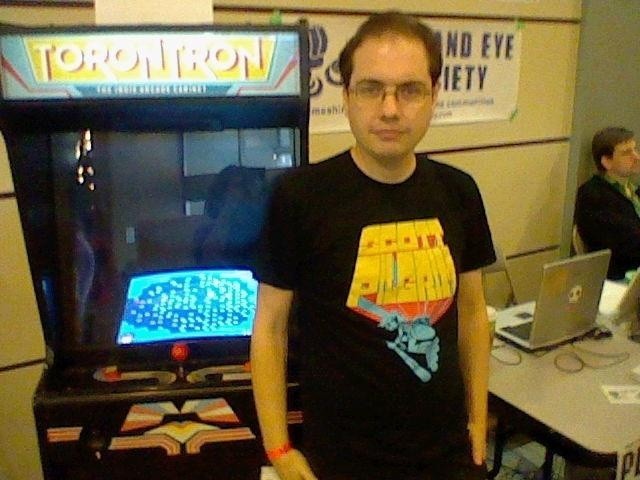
[189,164,275,259]
[571,121,640,290]
[243,7,500,480]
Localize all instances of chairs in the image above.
[482,246,519,310]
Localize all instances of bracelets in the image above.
[265,441,294,464]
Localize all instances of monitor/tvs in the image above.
[51,129,292,348]
[113,266,260,346]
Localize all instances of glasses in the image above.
[347,77,436,105]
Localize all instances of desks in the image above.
[487,277,639,480]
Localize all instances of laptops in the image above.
[495,246,613,354]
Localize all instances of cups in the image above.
[486,305,498,352]
